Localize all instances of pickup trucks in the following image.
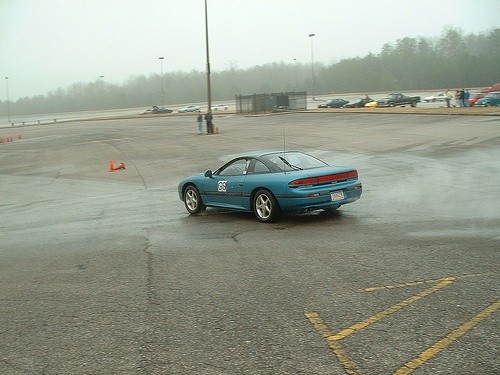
[376,93,421,108]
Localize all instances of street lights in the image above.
[308,33,315,98]
[5,77,8,121]
[158,56,164,108]
[99,75,104,91]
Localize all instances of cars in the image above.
[137,107,173,115]
[317,98,376,108]
[177,105,200,112]
[424,92,449,102]
[463,82,500,107]
[210,104,229,110]
[178,150,362,223]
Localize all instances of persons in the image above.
[204,110,213,135]
[197,111,203,134]
[454,88,470,107]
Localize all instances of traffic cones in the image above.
[108,159,114,172]
[114,163,126,171]
